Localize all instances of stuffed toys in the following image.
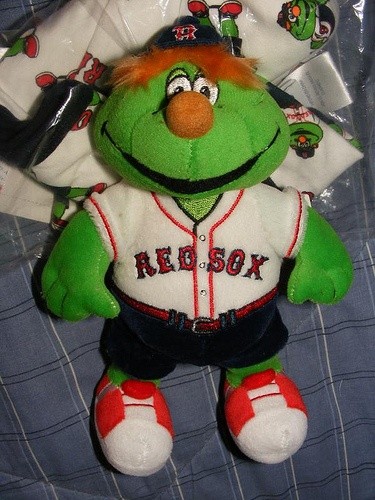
[37,15,356,477]
